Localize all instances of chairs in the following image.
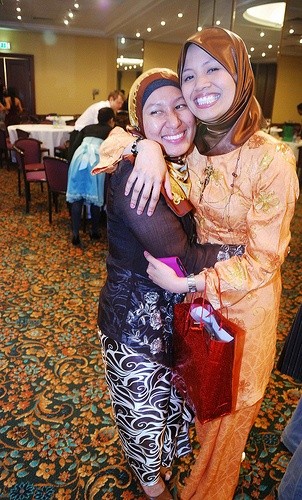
[0,116,112,246]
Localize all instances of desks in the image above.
[270,134,302,186]
[7,124,74,163]
